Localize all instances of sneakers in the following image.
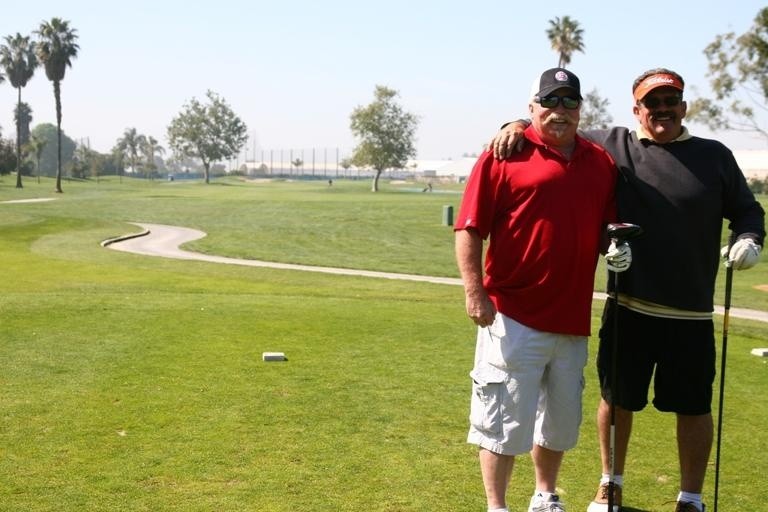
[674,501,706,511]
[587,481,622,512]
[528,494,567,512]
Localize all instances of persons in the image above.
[488,69,766,512]
[454,68,633,512]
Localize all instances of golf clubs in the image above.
[607,222,644,512]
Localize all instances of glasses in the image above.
[643,95,682,109]
[534,96,579,110]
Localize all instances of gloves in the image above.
[604,239,633,273]
[720,233,762,272]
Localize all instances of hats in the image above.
[535,68,584,101]
[632,68,685,104]
